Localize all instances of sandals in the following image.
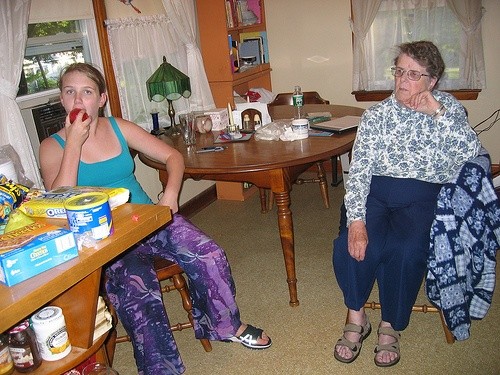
[220,319,272,349]
[374,319,400,367]
[333,318,372,363]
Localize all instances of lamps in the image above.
[145,56,191,136]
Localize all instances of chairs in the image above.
[268,91,330,210]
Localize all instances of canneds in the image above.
[0,306,72,375]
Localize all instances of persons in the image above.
[40,63,273,375]
[333,41,481,368]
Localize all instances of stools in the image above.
[103,261,213,367]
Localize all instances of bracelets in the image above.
[430,105,444,118]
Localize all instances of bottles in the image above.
[292,86,305,119]
[243,114,251,131]
[254,114,261,132]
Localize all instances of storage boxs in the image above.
[0,221,78,287]
[204,108,229,131]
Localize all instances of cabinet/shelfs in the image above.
[195,0,273,202]
[0,203,172,375]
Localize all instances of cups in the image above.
[240,56,257,68]
[179,111,196,145]
[291,119,311,139]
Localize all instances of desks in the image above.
[139,104,366,307]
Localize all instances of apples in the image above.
[69,109,89,124]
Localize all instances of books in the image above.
[225,0,265,64]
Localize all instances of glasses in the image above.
[390,65,429,80]
